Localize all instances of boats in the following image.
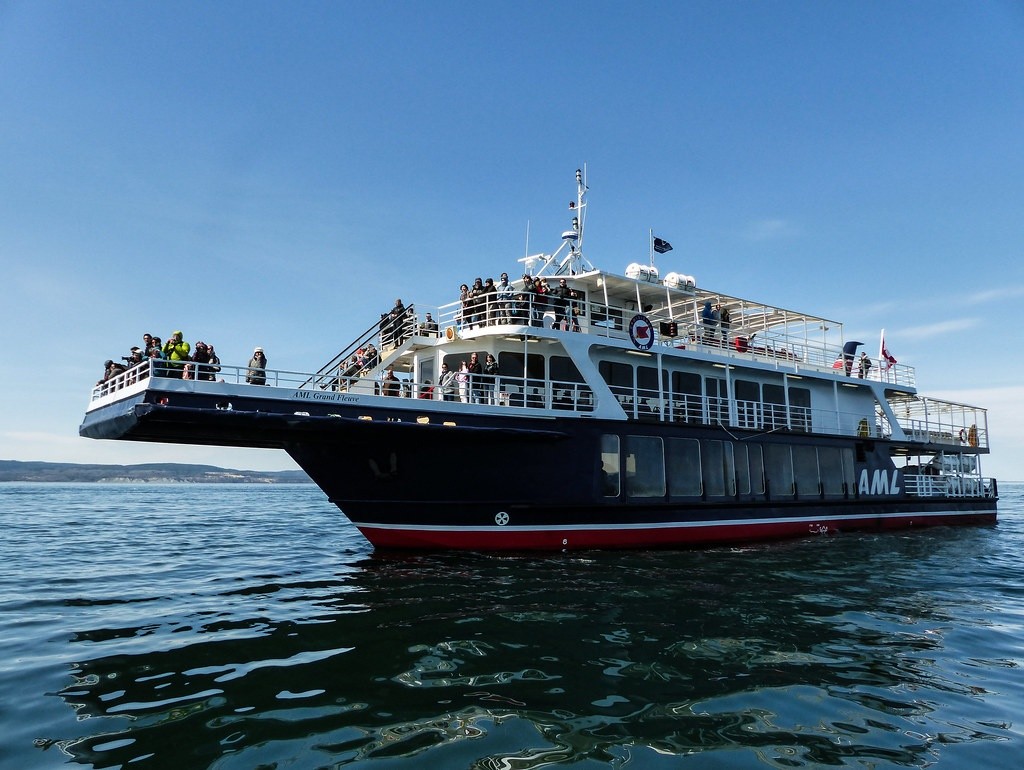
[81,161,1000,550]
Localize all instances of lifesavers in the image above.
[959,429,968,444]
[967,424,980,447]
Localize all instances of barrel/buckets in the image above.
[959,477,977,493]
[958,456,976,471]
[932,455,959,470]
[933,476,962,493]
[663,272,695,291]
[625,263,659,283]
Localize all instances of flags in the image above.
[653,235,673,253]
[881,334,897,370]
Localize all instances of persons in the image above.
[95,331,221,404]
[857,352,872,378]
[700,300,732,347]
[320,272,583,404]
[245,347,267,385]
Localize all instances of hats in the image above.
[134,349,144,357]
[104,360,113,367]
[254,347,263,354]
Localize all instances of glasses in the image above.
[472,357,477,359]
[560,282,565,283]
